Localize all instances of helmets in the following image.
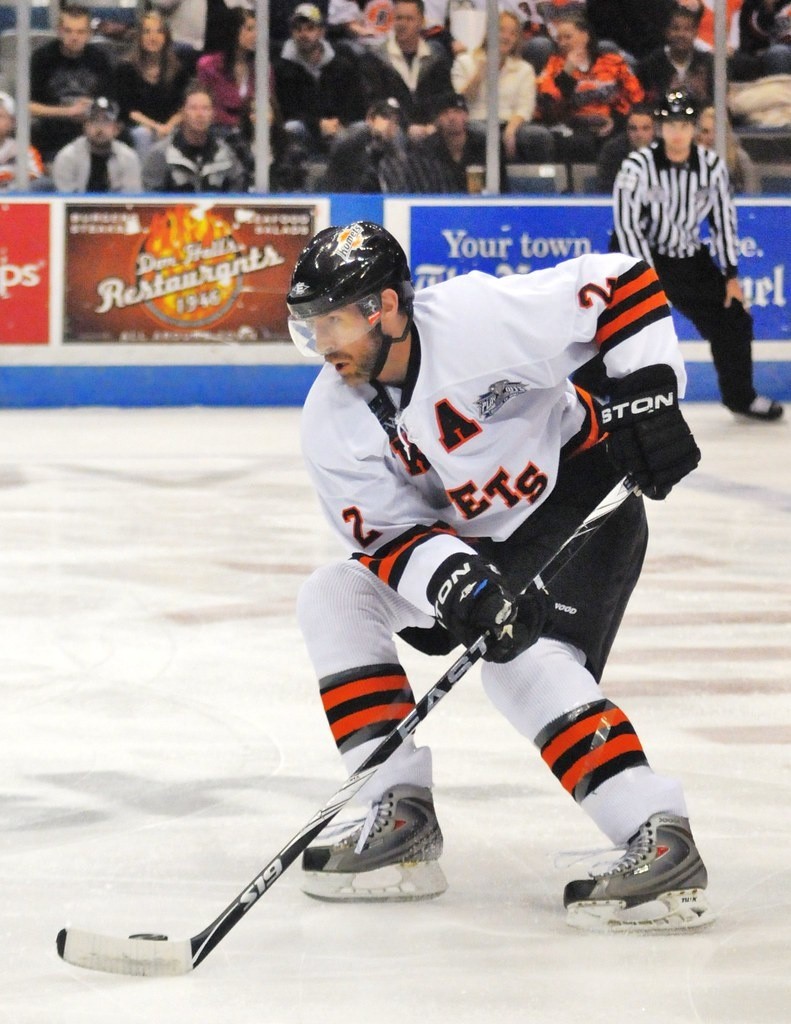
[652,87,698,122]
[286,220,414,317]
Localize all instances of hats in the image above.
[0,91,16,114]
[89,96,118,120]
[372,97,407,120]
[288,3,322,26]
[431,91,469,119]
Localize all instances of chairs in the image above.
[1,0,790,195]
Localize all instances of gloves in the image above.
[426,552,559,664]
[598,385,702,500]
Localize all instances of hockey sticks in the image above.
[53,468,641,978]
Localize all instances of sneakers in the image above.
[729,396,788,425]
[300,784,448,900]
[562,810,716,933]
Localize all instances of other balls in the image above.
[125,932,171,942]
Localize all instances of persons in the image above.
[611,85,784,422]
[311,98,512,195]
[281,220,709,931]
[105,7,187,150]
[141,89,246,194]
[52,96,144,195]
[31,6,116,162]
[0,93,47,193]
[453,0,791,197]
[197,6,284,173]
[272,3,364,152]
[356,1,470,140]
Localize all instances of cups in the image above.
[465,165,485,193]
